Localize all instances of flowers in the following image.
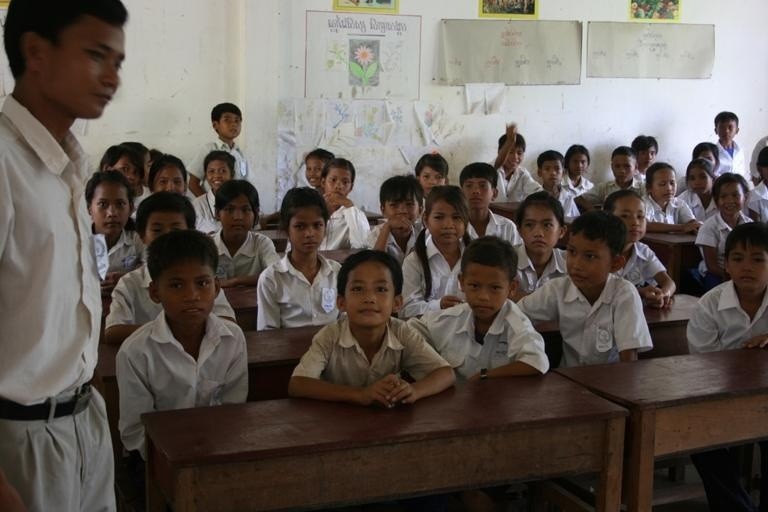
[349,44,379,86]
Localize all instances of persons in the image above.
[1,1,127,510]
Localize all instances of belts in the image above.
[0,383,93,420]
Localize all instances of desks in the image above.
[140,369,629,512]
[275,249,369,267]
[556,347,766,511]
[96,326,324,459]
[642,233,701,274]
[260,229,289,251]
[102,285,259,334]
[521,293,701,360]
